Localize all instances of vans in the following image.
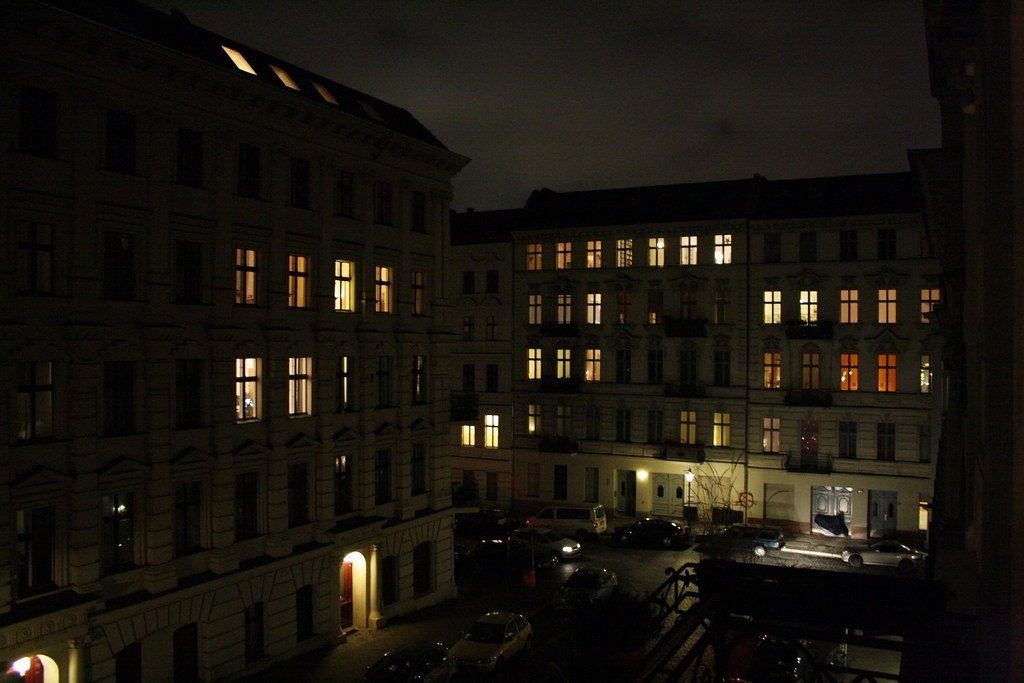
[527,502,608,544]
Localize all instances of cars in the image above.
[364,611,534,683]
[841,531,929,572]
[693,523,785,557]
[554,566,618,609]
[508,527,582,559]
[611,515,692,549]
[750,635,816,683]
